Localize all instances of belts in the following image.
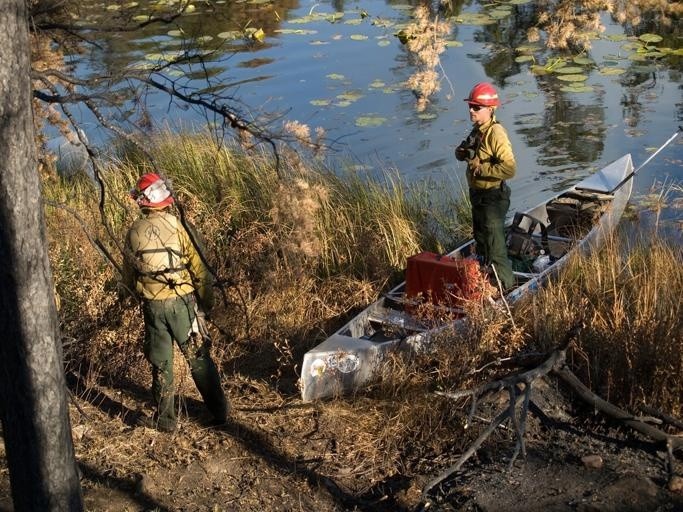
[469,188,501,194]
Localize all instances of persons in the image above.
[122,173,229,432]
[455,82,516,292]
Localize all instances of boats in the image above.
[302,154,634,401]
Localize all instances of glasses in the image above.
[469,105,484,111]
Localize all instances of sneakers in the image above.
[136,416,175,434]
[206,415,228,424]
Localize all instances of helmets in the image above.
[463,82,500,106]
[130,172,174,210]
